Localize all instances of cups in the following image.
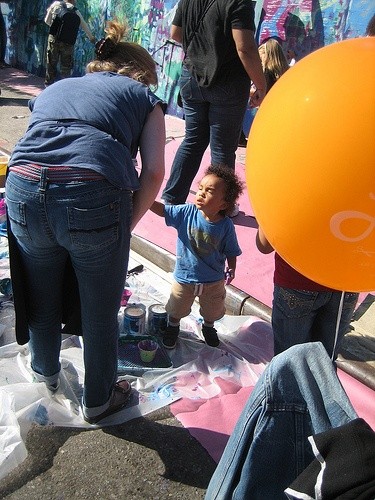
[138,340,159,363]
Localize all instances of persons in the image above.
[150,166,242,348]
[5,23,167,424]
[44,0,93,87]
[258,36,296,92]
[160,0,267,218]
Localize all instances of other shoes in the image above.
[202,323,219,347]
[18,349,60,393]
[162,324,180,349]
[83,381,132,422]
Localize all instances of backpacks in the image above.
[55,1,81,46]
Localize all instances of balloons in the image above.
[245,36,375,291]
